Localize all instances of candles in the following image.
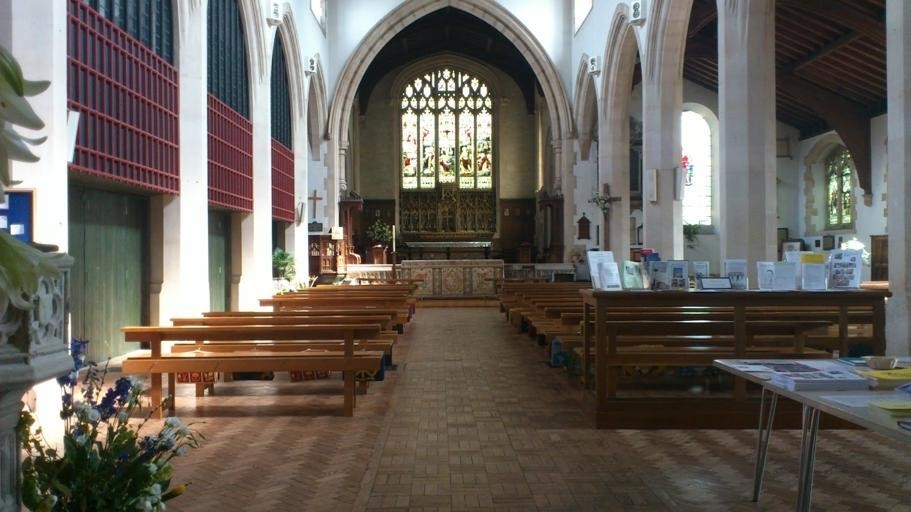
[392,223,397,253]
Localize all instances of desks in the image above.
[710,353,911,511]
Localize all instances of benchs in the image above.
[121,277,425,420]
[484,277,893,431]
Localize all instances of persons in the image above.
[398,143,491,173]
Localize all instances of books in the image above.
[729,354,911,432]
[585,249,865,292]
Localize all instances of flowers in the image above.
[17,329,209,510]
